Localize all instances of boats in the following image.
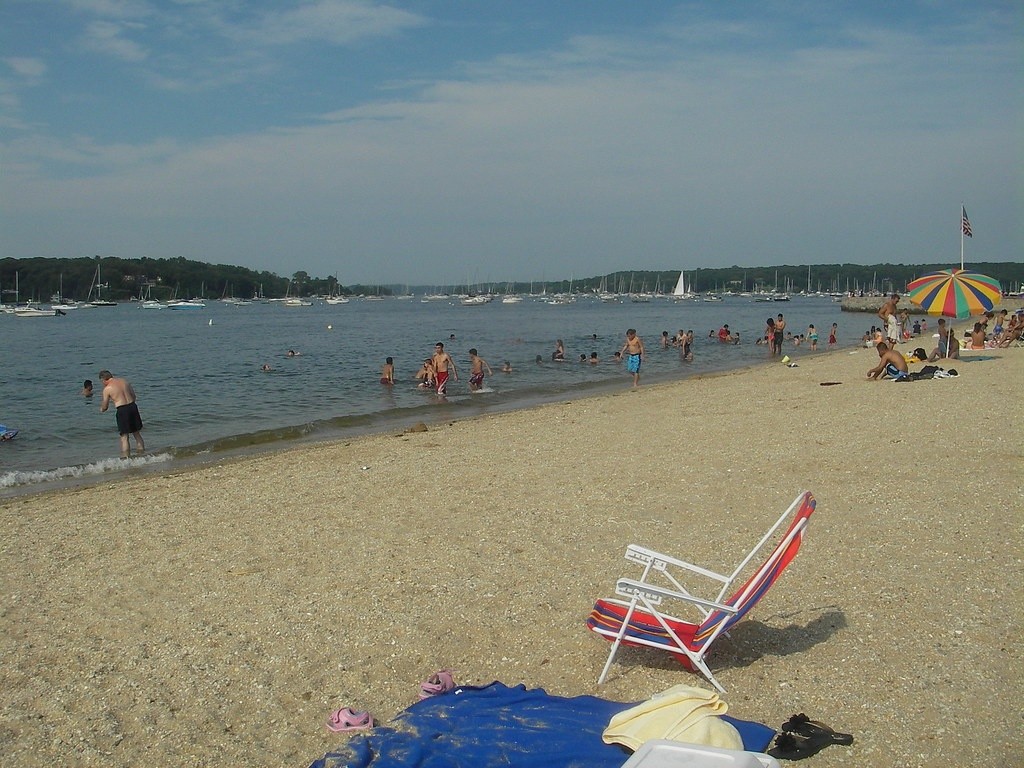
[14,300,67,316]
[1001,281,1024,296]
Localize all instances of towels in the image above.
[887,313,898,340]
[601,683,745,752]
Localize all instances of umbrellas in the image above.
[908,269,1001,358]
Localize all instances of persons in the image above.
[929,308,1024,361]
[862,308,926,348]
[867,342,908,381]
[98,371,146,451]
[755,313,808,356]
[553,339,565,361]
[467,348,492,392]
[416,342,457,397]
[710,324,740,346]
[287,350,296,356]
[578,354,586,363]
[449,334,456,342]
[620,329,643,387]
[807,324,817,353]
[879,294,901,350]
[502,361,512,373]
[612,352,621,362]
[589,352,599,363]
[661,329,694,361]
[82,380,94,397]
[262,364,271,371]
[380,357,395,386]
[829,323,838,349]
[592,333,597,341]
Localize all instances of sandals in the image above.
[419,670,458,700]
[325,707,381,732]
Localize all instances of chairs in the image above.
[1012,331,1024,349]
[586,490,816,694]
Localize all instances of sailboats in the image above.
[0,264,118,314]
[130,264,910,310]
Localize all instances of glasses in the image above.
[435,347,440,349]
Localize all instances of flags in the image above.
[960,207,973,239]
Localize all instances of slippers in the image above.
[767,732,834,761]
[782,712,854,745]
